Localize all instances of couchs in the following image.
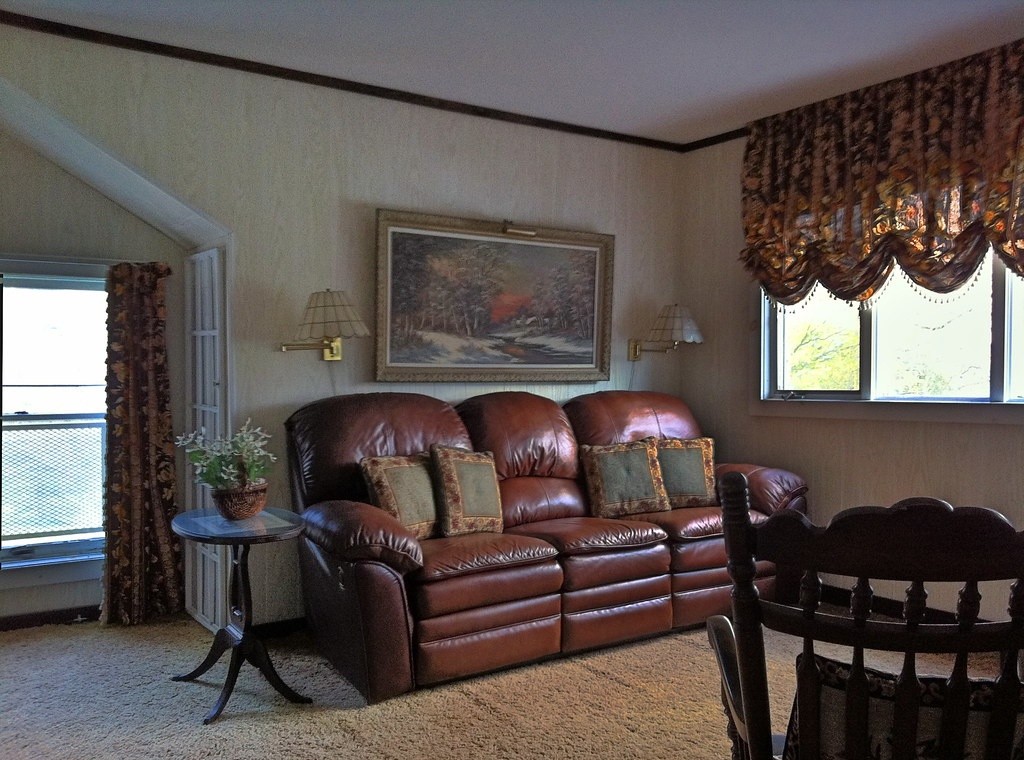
[283,390,808,705]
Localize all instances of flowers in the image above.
[175,417,279,489]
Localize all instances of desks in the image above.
[171,505,313,726]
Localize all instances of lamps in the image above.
[628,303,705,363]
[281,288,373,362]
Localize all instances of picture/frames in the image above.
[374,208,616,382]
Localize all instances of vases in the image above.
[209,477,267,522]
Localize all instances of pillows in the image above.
[579,435,672,518]
[657,437,719,509]
[428,442,504,536]
[361,450,441,540]
[781,652,1024,760]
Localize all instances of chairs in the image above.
[706,471,1024,760]
